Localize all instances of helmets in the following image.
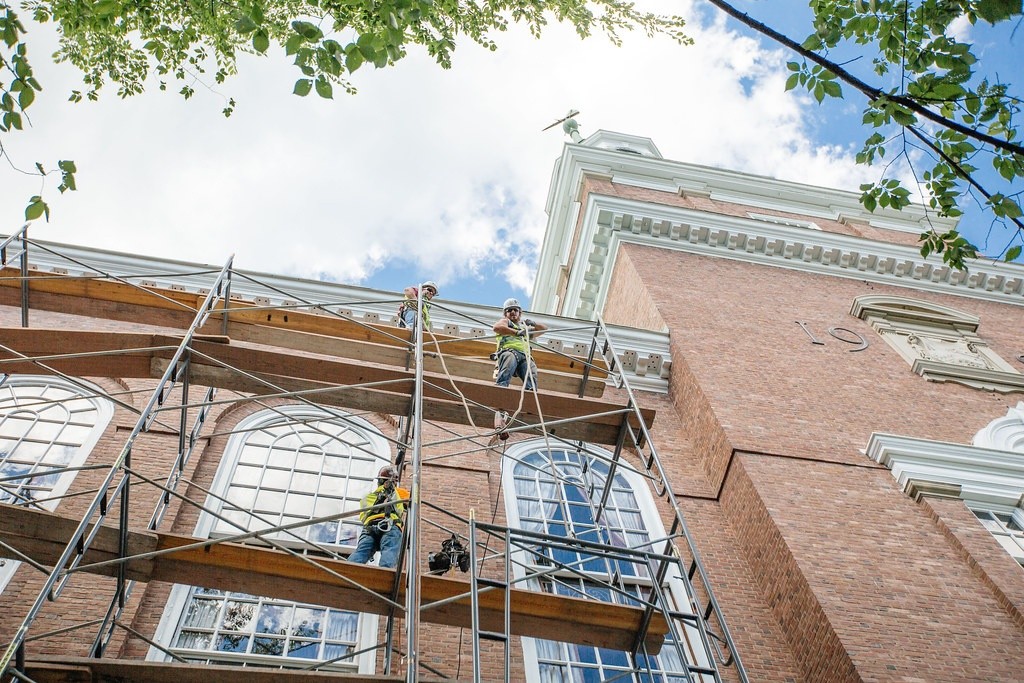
[503,299,522,316]
[422,281,439,296]
[374,466,399,480]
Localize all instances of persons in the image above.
[492,299,549,390]
[347,464,411,568]
[401,280,440,330]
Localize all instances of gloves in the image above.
[521,319,537,326]
[516,329,526,336]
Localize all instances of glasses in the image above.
[427,287,435,296]
[506,309,519,312]
